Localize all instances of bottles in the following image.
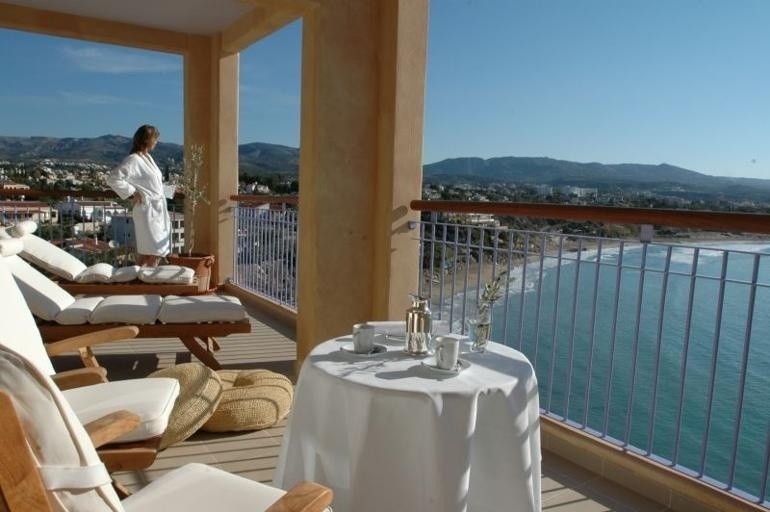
[93,234,99,244]
[405,294,432,354]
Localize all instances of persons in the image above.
[106,124,189,266]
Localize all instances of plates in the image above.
[340,344,390,358]
[421,355,470,375]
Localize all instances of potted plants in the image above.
[470,263,518,354]
[165,143,216,292]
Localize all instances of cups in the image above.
[350,323,374,352]
[436,335,460,372]
